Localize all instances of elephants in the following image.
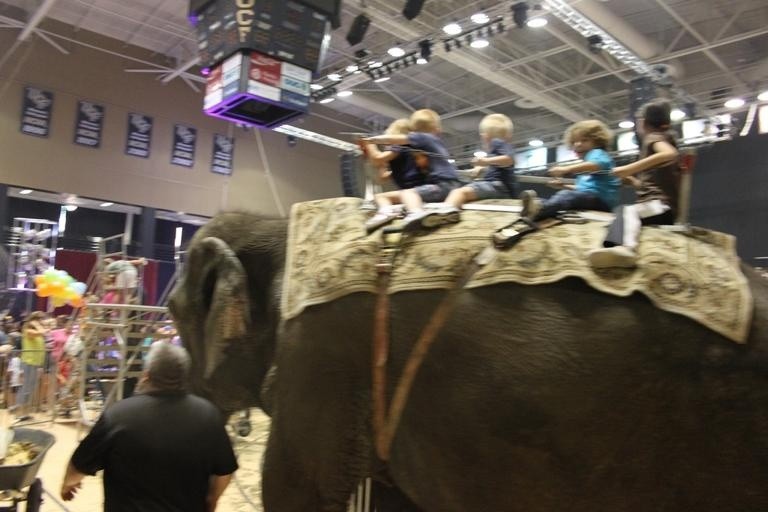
[166,207,768,511]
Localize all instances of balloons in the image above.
[33,267,89,310]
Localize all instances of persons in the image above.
[419,113,517,229]
[587,101,681,267]
[353,109,461,234]
[1,312,81,417]
[520,119,621,223]
[60,341,237,511]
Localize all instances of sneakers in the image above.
[590,242,635,270]
[520,189,540,220]
[402,207,460,227]
[365,208,396,230]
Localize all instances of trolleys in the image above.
[0,427,55,512]
